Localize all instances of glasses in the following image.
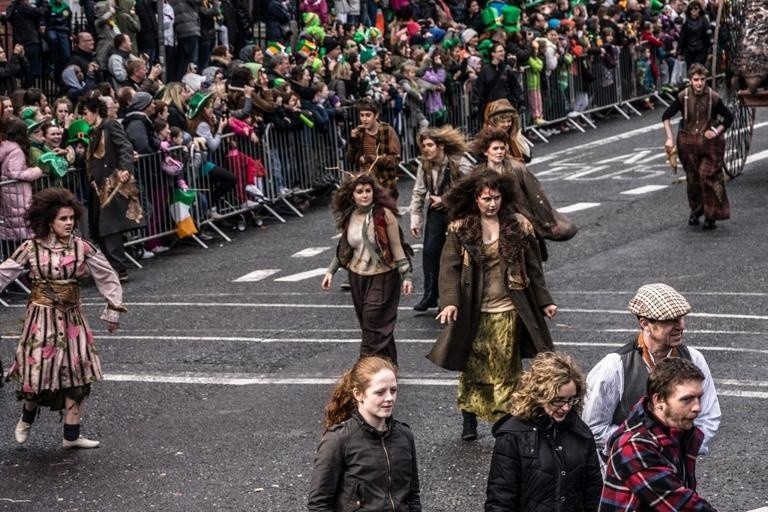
[549,397,581,407]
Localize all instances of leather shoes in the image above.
[703,219,714,229]
[688,215,699,226]
[14,413,36,444]
[61,434,100,450]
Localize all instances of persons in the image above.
[428,127,557,262]
[306,356,421,512]
[484,351,603,512]
[424,167,558,440]
[580,283,722,482]
[463,98,534,162]
[321,174,413,368]
[598,358,718,512]
[409,124,475,311]
[329,102,414,289]
[661,62,733,229]
[0,1,763,289]
[0,187,127,449]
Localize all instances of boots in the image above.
[414,271,437,311]
[462,408,477,440]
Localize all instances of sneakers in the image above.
[139,249,154,258]
[246,185,263,197]
[527,117,597,140]
[241,200,258,208]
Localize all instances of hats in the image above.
[628,283,692,322]
[487,98,516,119]
[302,6,521,54]
[21,92,215,145]
